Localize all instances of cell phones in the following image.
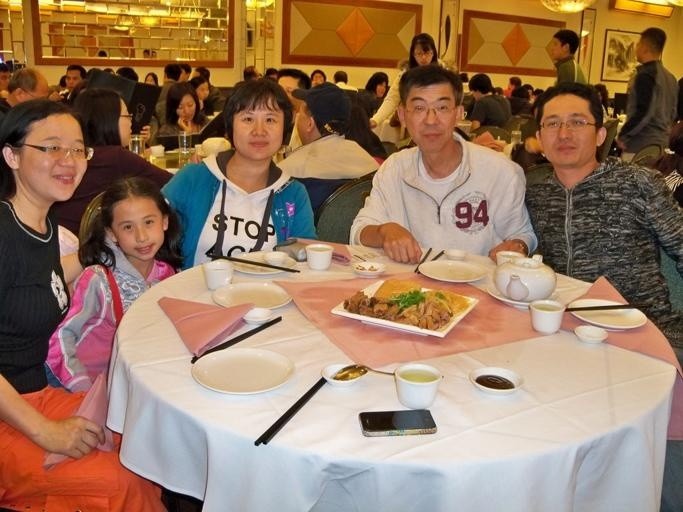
[358,409,437,437]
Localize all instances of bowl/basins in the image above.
[441,250,466,261]
[321,361,370,389]
[353,261,384,278]
[200,136,232,155]
[262,252,289,267]
[242,307,273,327]
[574,324,609,344]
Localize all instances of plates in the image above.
[211,280,291,311]
[330,278,480,341]
[232,249,297,276]
[417,261,491,283]
[567,297,650,334]
[468,366,524,398]
[484,284,562,311]
[192,347,295,396]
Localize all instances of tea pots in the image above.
[492,254,558,303]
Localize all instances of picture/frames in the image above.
[601,30,641,81]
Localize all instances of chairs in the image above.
[544,0,598,13]
[660,253,683,320]
[316,172,375,244]
[77,190,107,244]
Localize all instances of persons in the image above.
[370,33,453,128]
[523,85,532,93]
[276,69,311,145]
[0,69,52,122]
[358,72,388,118]
[506,87,533,115]
[263,69,276,84]
[494,88,509,102]
[334,71,359,92]
[144,73,159,86]
[525,83,682,369]
[311,71,327,87]
[177,64,192,81]
[159,80,319,274]
[345,104,389,167]
[529,89,544,106]
[47,65,87,101]
[44,177,190,392]
[191,68,210,80]
[1,100,171,512]
[244,65,262,81]
[592,83,611,125]
[55,88,174,283]
[116,66,139,81]
[350,66,538,264]
[48,75,67,98]
[275,82,381,217]
[469,74,513,129]
[0,63,11,100]
[156,82,211,137]
[190,76,214,117]
[503,77,522,100]
[102,67,117,77]
[552,29,589,86]
[617,28,678,166]
[161,63,181,89]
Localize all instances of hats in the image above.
[293,83,349,127]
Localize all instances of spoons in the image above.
[332,363,399,383]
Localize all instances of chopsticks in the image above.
[414,247,445,276]
[230,252,301,274]
[253,376,327,446]
[191,315,284,365]
[562,302,653,315]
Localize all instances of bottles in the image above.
[129,135,144,159]
[511,130,521,147]
[178,131,191,169]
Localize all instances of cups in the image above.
[495,250,524,268]
[528,300,565,335]
[177,130,193,163]
[128,135,146,160]
[393,364,443,407]
[206,259,235,290]
[510,130,524,148]
[304,243,334,271]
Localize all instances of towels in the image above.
[42,372,115,469]
[291,237,352,264]
[559,276,682,377]
[273,273,560,372]
[158,298,253,357]
[473,130,506,152]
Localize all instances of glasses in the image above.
[17,143,94,161]
[540,117,594,131]
[22,88,35,98]
[120,114,135,121]
[414,50,432,57]
[404,104,459,116]
